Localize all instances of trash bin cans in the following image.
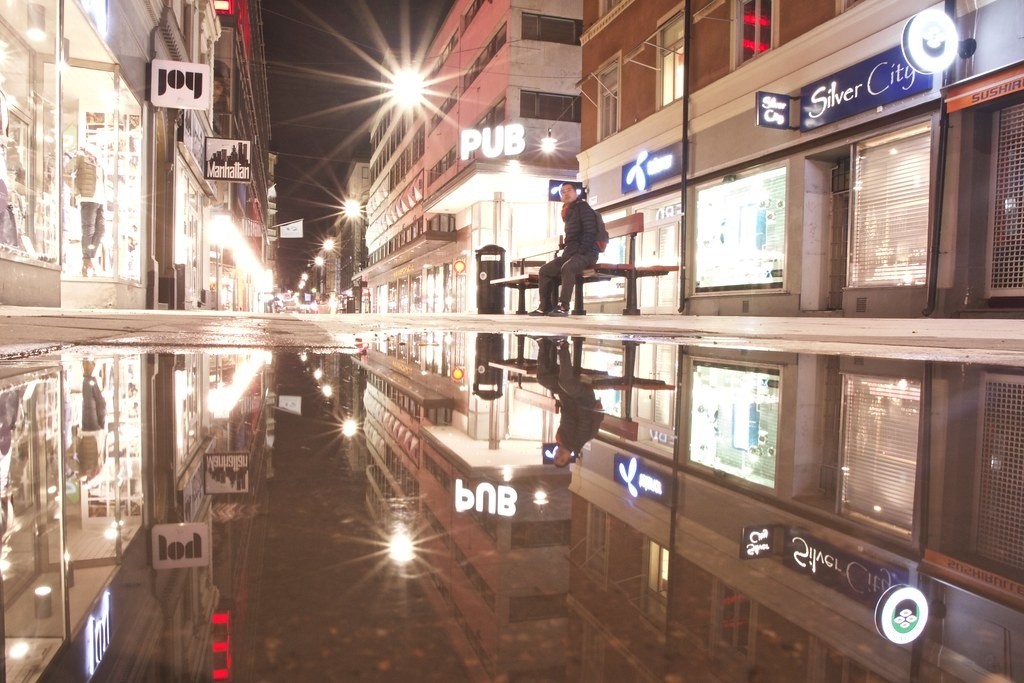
[474,245,507,316]
[473,332,505,401]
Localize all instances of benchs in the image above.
[487,335,676,442]
[489,211,680,315]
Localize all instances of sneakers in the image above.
[549,302,569,316]
[529,306,549,315]
[548,336,569,351]
[528,336,550,344]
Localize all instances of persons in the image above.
[529,181,601,318]
[534,338,605,468]
[64,145,109,277]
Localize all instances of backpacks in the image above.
[573,201,609,253]
[574,400,606,453]
